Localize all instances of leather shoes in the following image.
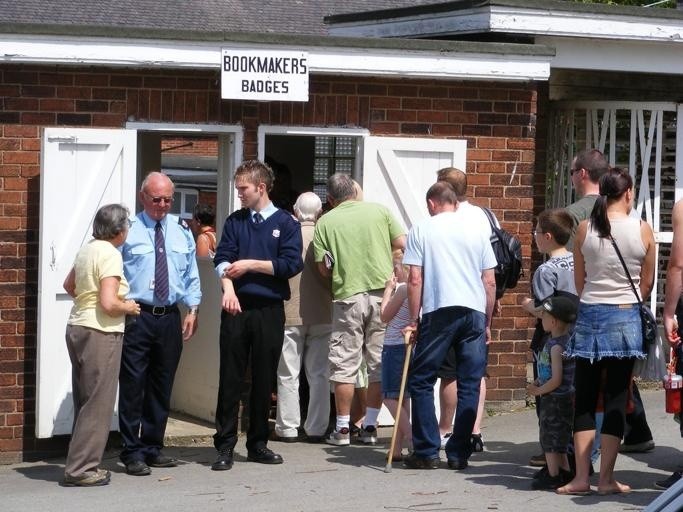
[247,448,283,463]
[211,449,234,470]
[128,461,151,476]
[150,452,178,466]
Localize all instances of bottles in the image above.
[663,373,683,414]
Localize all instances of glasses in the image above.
[144,192,171,203]
[570,167,581,175]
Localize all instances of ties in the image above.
[154,223,169,302]
[253,214,262,224]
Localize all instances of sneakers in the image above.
[326,430,351,446]
[529,454,573,490]
[356,424,377,444]
[62,469,111,487]
[619,440,656,453]
[403,453,440,470]
[448,459,468,470]
[471,433,484,452]
[654,470,683,490]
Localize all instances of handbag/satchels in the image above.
[640,303,657,346]
[491,225,523,300]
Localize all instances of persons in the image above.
[62,149,683,497]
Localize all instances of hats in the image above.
[535,296,577,324]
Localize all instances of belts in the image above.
[138,303,176,318]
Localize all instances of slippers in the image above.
[556,485,593,496]
[598,484,633,495]
